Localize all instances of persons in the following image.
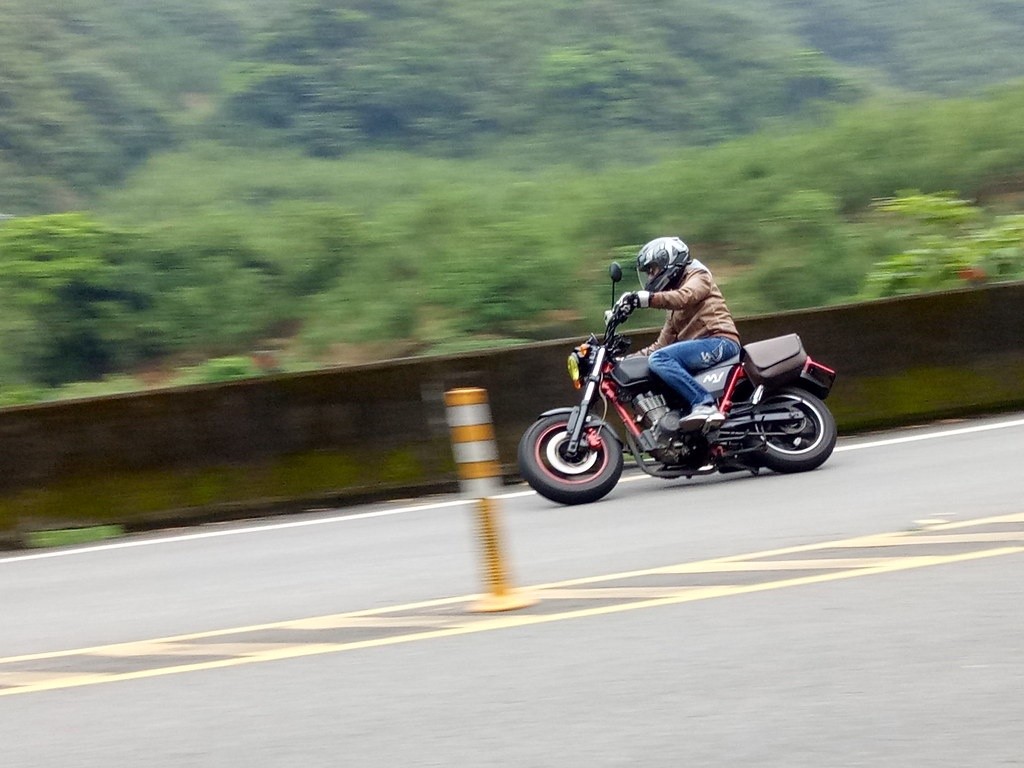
[612,235,743,432]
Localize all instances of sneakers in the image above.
[679,404,725,430]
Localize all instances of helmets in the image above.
[636,235,689,293]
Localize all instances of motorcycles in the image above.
[517,261,837,507]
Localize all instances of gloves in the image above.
[612,288,650,317]
